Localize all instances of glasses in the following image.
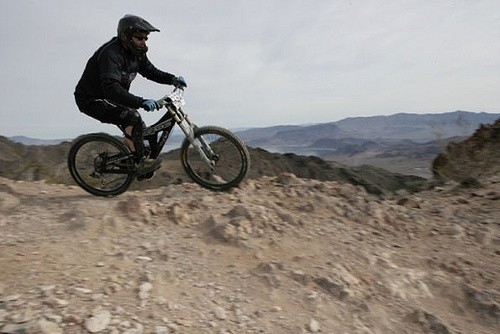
[130,34,148,41]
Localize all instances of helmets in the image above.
[117,16,160,57]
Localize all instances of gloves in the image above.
[172,76,187,91]
[139,99,160,112]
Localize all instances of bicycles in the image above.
[67,84,251,198]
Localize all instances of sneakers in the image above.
[138,171,153,181]
[137,158,161,175]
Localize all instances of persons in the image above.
[74,15,187,181]
